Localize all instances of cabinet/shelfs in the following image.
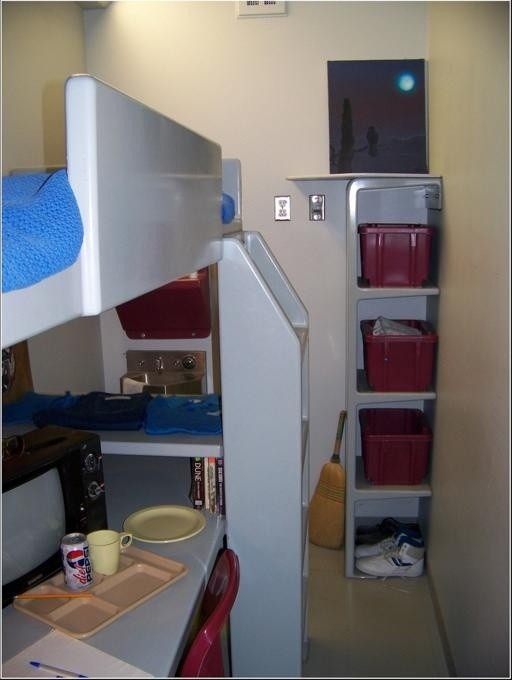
[345,178,445,578]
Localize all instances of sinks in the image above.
[132,371,197,386]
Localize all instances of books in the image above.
[3,628,154,678]
[188,456,226,521]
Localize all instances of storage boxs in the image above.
[358,223,433,288]
[360,319,437,392]
[358,406,432,485]
[115,266,211,339]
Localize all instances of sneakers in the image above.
[355,537,425,577]
[354,527,422,558]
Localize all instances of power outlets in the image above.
[274,195,290,220]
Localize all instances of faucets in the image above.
[155,357,165,373]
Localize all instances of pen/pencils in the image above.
[14,593,95,598]
[30,662,88,679]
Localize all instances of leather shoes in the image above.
[355,524,421,545]
[357,517,419,535]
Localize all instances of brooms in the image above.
[306,409,345,552]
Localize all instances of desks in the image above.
[1,454,226,680]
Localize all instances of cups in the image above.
[86,528,132,578]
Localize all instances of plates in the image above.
[125,504,205,546]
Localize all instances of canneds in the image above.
[60,532,94,591]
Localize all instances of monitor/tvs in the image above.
[2,425,109,610]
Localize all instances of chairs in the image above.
[176,547,241,677]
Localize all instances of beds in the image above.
[2,75,242,348]
